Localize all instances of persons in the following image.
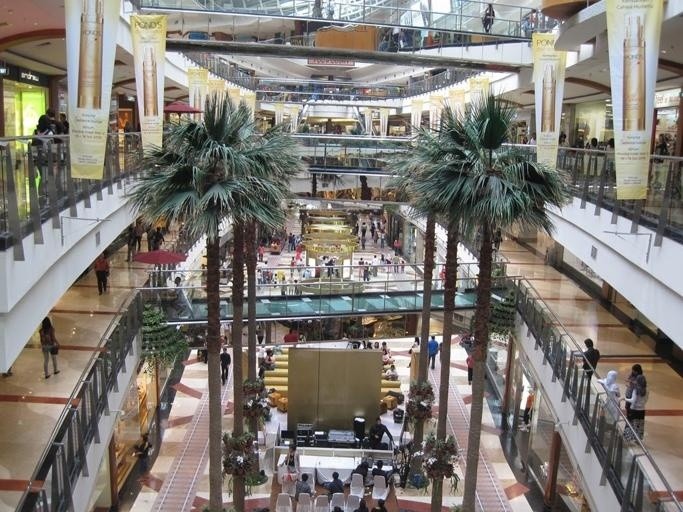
[257,255,339,294]
[306,187,400,202]
[520,388,535,432]
[339,211,386,250]
[134,433,153,473]
[376,27,423,52]
[258,83,359,103]
[30,108,69,196]
[201,335,232,387]
[105,122,141,166]
[258,349,275,381]
[359,239,404,282]
[466,353,474,384]
[126,217,189,262]
[351,340,398,380]
[255,228,304,261]
[654,132,677,165]
[268,116,411,147]
[39,317,60,379]
[523,132,615,177]
[169,276,187,316]
[280,407,410,510]
[583,339,648,446]
[408,336,443,371]
[94,253,109,295]
[478,226,503,252]
[256,320,374,344]
[481,5,495,33]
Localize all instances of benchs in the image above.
[254,339,404,416]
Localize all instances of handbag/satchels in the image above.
[50,346,59,356]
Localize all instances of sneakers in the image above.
[45,374,51,379]
[54,371,60,375]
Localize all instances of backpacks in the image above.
[30,129,51,163]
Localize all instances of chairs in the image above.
[220,376,456,511]
